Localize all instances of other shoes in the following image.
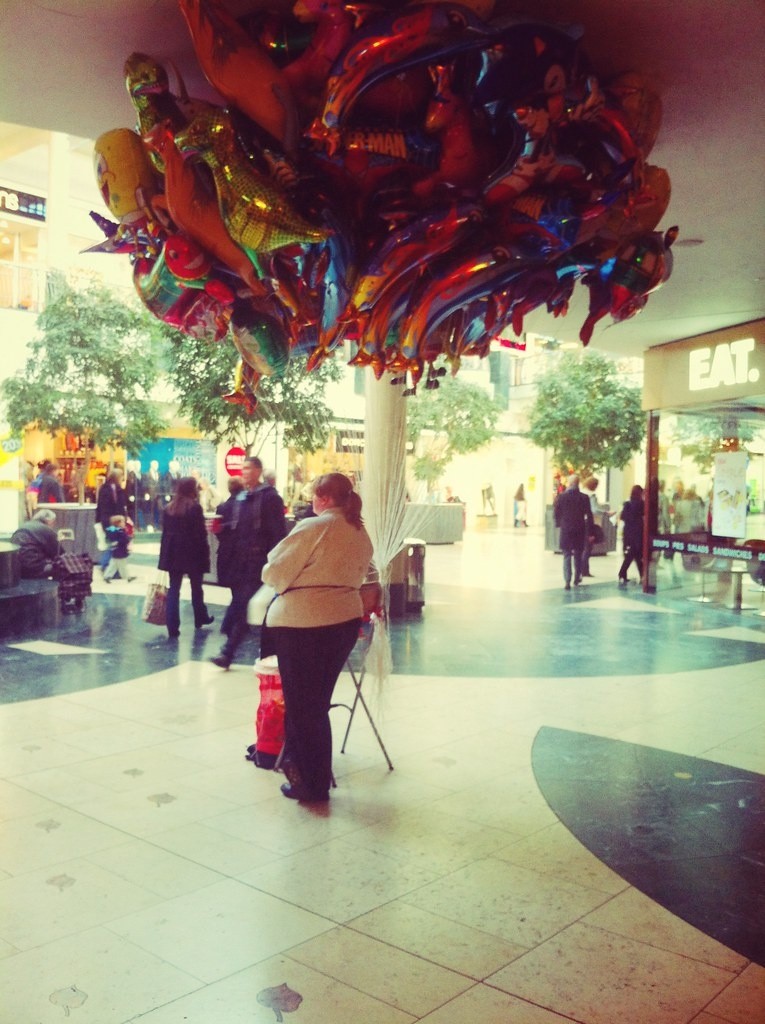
[208,652,231,668]
[574,578,582,585]
[104,577,111,583]
[281,783,297,798]
[582,573,594,578]
[128,576,136,581]
[565,585,571,589]
[168,627,180,636]
[195,615,214,628]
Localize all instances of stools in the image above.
[0,574,59,644]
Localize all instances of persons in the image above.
[24,459,66,519]
[208,456,286,669]
[260,473,372,803]
[263,469,318,523]
[618,485,646,582]
[159,475,214,639]
[103,516,137,582]
[553,474,593,591]
[656,480,707,561]
[581,476,616,577]
[442,485,460,503]
[95,468,127,580]
[513,479,529,528]
[126,460,181,532]
[215,477,250,634]
[10,510,66,581]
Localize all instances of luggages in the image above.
[52,528,93,613]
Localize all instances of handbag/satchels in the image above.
[142,583,170,625]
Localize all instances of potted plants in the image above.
[2,283,164,566]
[162,309,344,586]
[527,345,649,556]
[391,355,501,545]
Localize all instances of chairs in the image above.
[273,581,396,787]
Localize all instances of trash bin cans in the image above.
[391,541,425,619]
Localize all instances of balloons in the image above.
[82,1,679,416]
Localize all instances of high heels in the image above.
[619,572,630,581]
